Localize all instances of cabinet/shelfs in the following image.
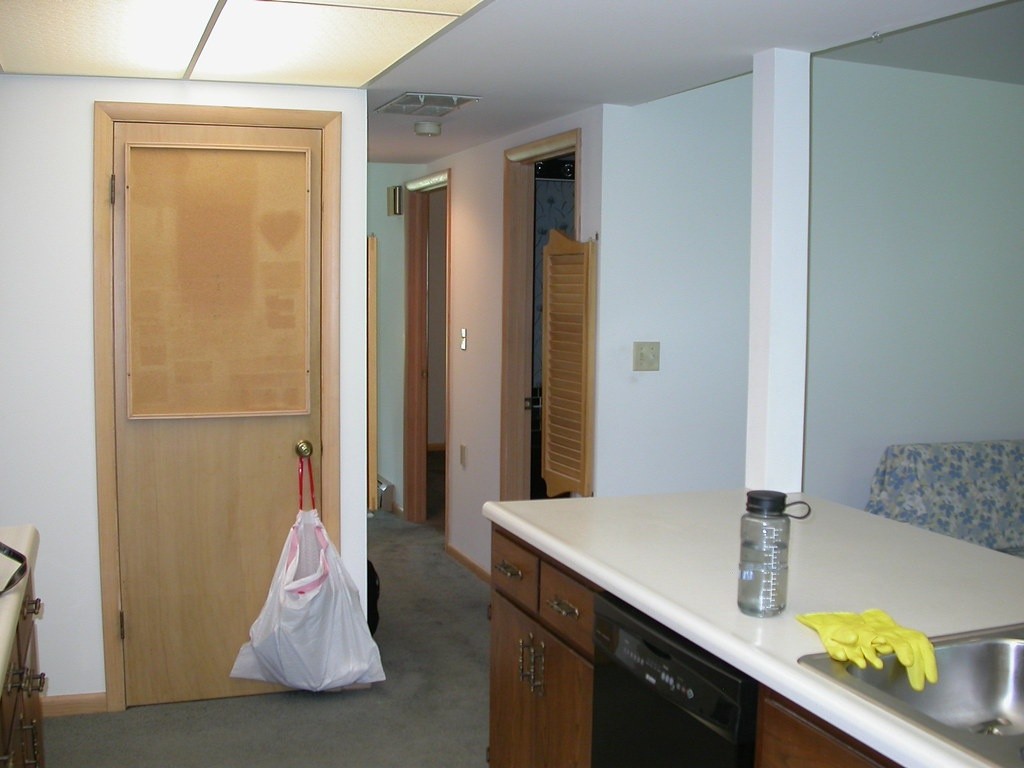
[0,569,48,768]
[486,522,597,768]
[756,685,906,768]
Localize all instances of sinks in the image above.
[797,618,1024,768]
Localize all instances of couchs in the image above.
[864,440,1024,553]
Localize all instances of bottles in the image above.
[739,492,810,619]
